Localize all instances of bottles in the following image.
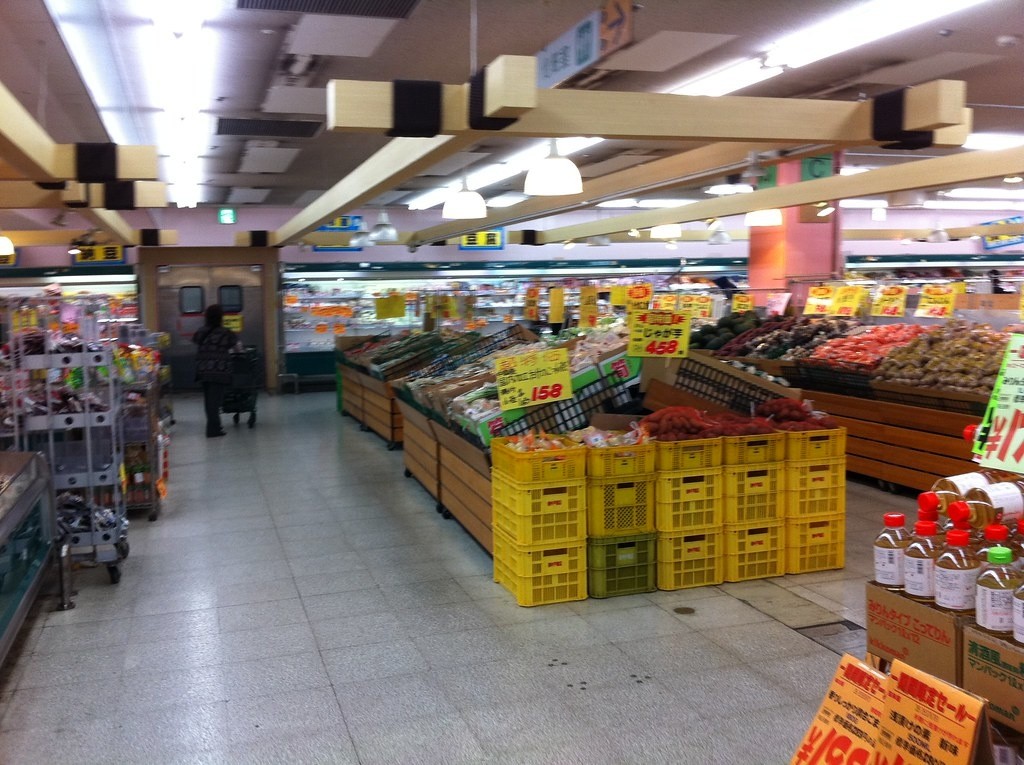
[872,424,1024,649]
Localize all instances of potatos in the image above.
[638,396,838,441]
[872,318,1009,395]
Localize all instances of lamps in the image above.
[349,222,375,247]
[369,211,398,241]
[440,149,488,219]
[523,139,585,196]
[0,236,15,254]
[50,211,103,254]
[588,148,924,248]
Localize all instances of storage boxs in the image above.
[491,428,852,608]
[862,578,1024,734]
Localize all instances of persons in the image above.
[191,303,242,438]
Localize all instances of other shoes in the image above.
[206,425,227,437]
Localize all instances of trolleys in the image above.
[8,295,130,585]
[216,351,261,428]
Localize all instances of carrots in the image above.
[808,325,938,375]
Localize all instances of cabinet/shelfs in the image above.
[0,296,171,583]
[280,260,1024,382]
